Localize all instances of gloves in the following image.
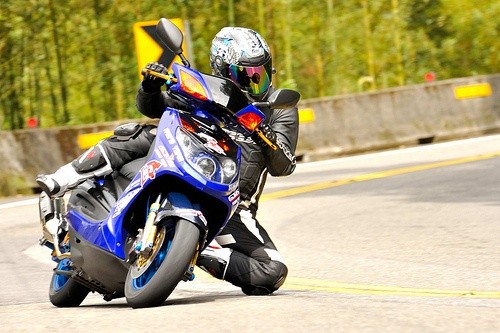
[143,62,168,84]
[252,122,276,147]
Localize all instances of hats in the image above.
[209,26,272,96]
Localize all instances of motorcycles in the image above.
[38,17,301,308]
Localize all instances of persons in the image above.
[36,27,299,296]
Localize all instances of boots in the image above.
[36,143,114,198]
[197,242,232,280]
